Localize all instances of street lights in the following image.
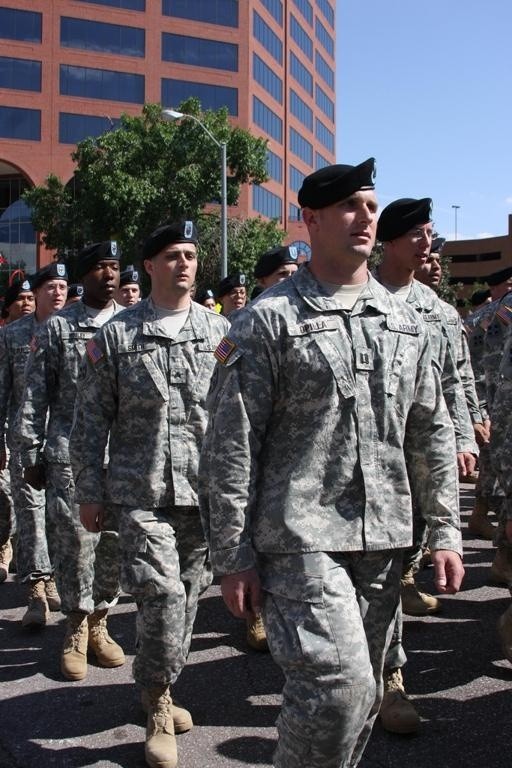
[451,204,462,243]
[161,107,230,282]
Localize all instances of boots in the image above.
[137,685,195,767]
[20,580,51,631]
[489,540,512,662]
[398,542,446,616]
[468,498,502,540]
[44,575,63,612]
[59,611,90,680]
[241,601,271,650]
[0,536,15,585]
[379,662,424,740]
[87,607,128,670]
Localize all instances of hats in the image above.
[451,264,512,307]
[71,240,122,279]
[4,275,34,306]
[142,218,201,259]
[375,196,434,242]
[197,290,215,304]
[66,283,85,298]
[253,243,300,279]
[215,271,247,295]
[295,157,380,211]
[118,270,141,286]
[33,260,69,286]
[430,237,447,254]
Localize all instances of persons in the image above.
[370,191,484,739]
[194,155,467,767]
[1,227,511,683]
[67,215,249,767]
